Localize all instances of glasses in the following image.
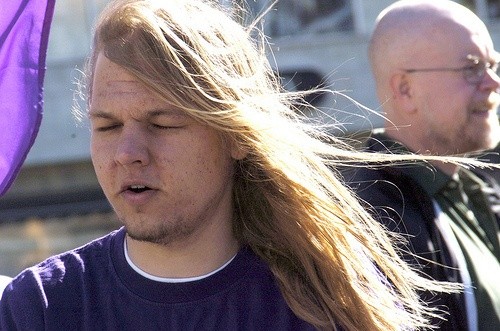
[405,59,500,84]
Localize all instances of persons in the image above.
[347,1,500,331]
[1,0,499,331]
